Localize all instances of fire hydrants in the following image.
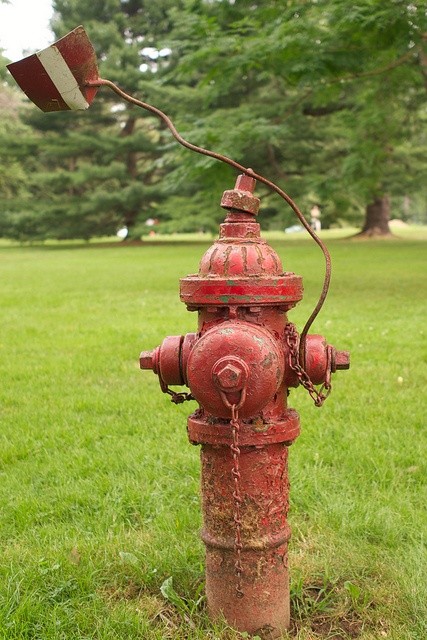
[137,165,350,640]
